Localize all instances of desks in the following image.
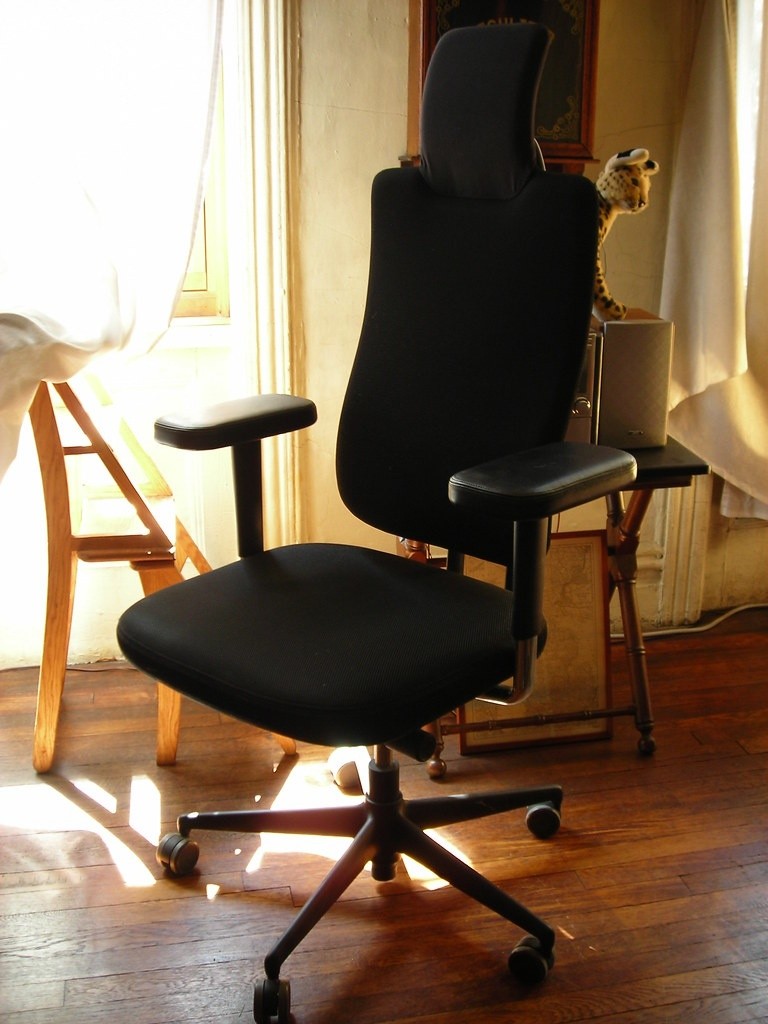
[421,432,711,778]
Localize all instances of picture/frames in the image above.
[456,526,615,754]
[398,0,602,176]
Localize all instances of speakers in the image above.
[594,307,675,450]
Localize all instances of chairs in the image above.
[0,313,297,774]
[115,18,639,1024]
